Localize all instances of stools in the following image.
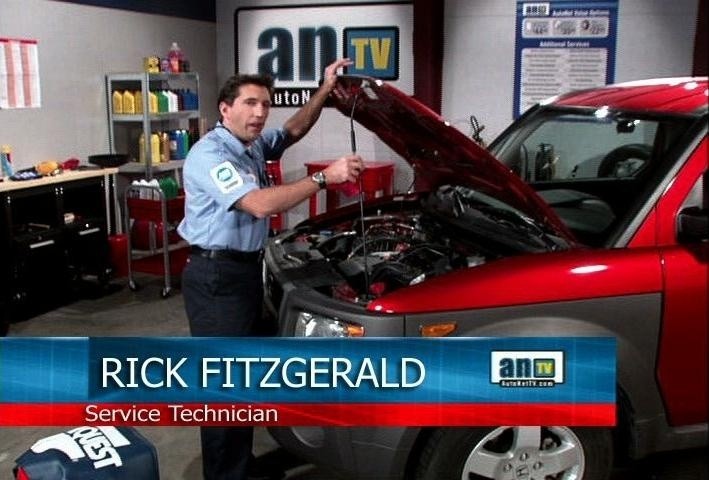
[12,426,161,480]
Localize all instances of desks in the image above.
[305,161,394,217]
[0,166,120,336]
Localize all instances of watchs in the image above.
[311,172,328,190]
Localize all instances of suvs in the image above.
[258,73,709,480]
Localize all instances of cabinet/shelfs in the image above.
[105,70,203,170]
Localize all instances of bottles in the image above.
[1,145,13,181]
[169,42,183,71]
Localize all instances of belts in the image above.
[190,246,266,264]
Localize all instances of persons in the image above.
[176,56,365,480]
